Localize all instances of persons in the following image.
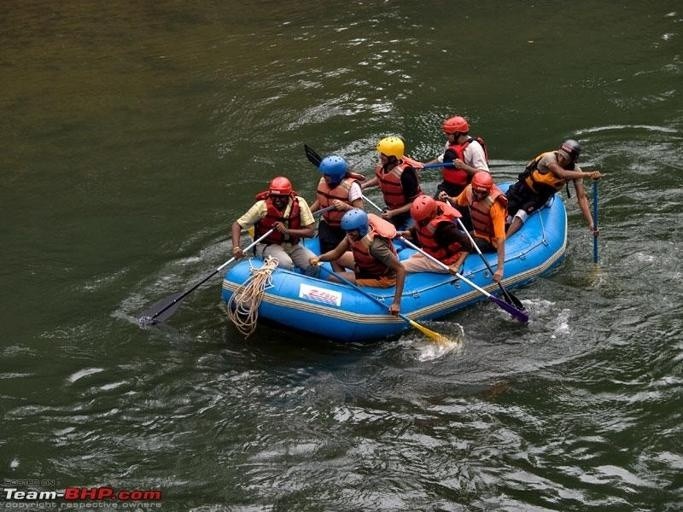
[503,139,600,238]
[307,156,364,254]
[231,176,320,280]
[308,207,406,315]
[398,193,473,276]
[412,116,490,233]
[437,171,508,284]
[360,136,423,248]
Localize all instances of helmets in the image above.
[558,140,582,160]
[410,195,437,221]
[340,208,368,237]
[472,172,493,193]
[269,177,292,195]
[443,116,469,134]
[376,136,405,160]
[319,155,347,185]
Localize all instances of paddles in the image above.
[305,145,386,213]
[399,236,529,324]
[141,226,275,324]
[442,196,525,313]
[315,263,453,348]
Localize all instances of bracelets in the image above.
[232,246,239,251]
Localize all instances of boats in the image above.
[221,181,568,342]
